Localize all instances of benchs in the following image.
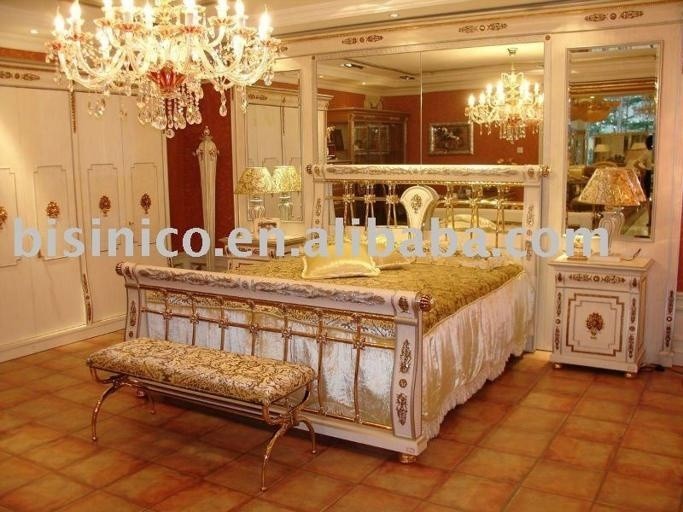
[84,339,319,492]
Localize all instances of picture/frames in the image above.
[426,122,473,155]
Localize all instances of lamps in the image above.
[614,168,646,242]
[577,169,639,256]
[45,0,283,139]
[592,144,608,162]
[462,48,544,144]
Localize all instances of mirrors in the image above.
[558,41,662,243]
[311,34,544,252]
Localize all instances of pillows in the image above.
[300,234,410,279]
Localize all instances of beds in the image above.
[115,164,548,466]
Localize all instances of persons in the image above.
[633,135,653,227]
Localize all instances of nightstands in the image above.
[218,228,310,270]
[545,249,654,379]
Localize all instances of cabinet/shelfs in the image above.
[246,84,333,237]
[0,55,172,362]
[327,106,408,225]
